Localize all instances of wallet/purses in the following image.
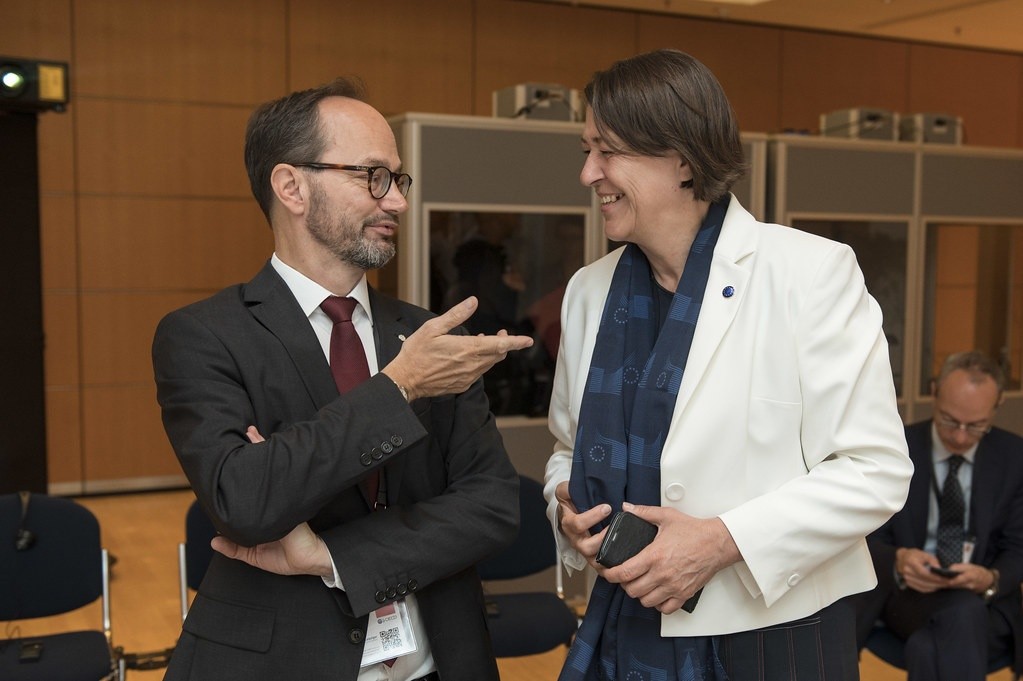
[596,512,704,613]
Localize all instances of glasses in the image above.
[936,389,999,438]
[292,163,412,199]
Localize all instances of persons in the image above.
[542,49,914,681]
[859,350,1023,681]
[150,83,534,681]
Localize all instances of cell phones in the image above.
[930,567,957,580]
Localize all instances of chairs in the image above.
[483,475,577,658]
[0,494,125,681]
[179,496,216,624]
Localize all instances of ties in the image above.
[929,455,966,578]
[319,297,400,668]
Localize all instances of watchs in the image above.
[981,569,999,599]
